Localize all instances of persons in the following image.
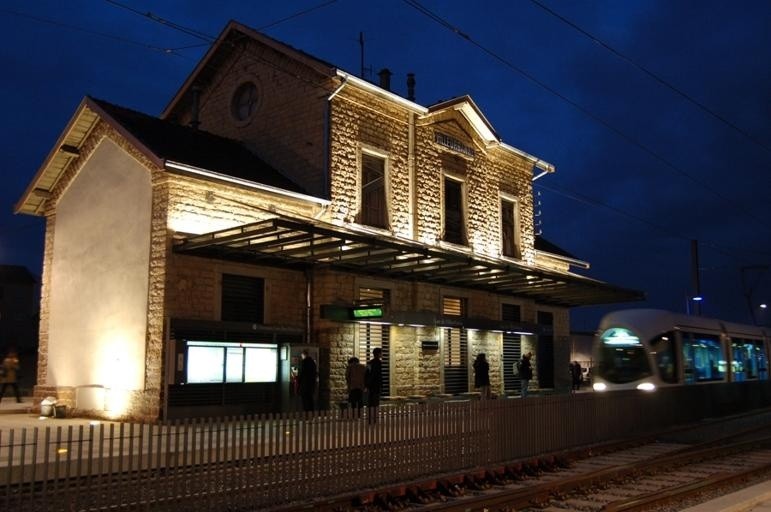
[299,350,318,411]
[569,361,582,390]
[472,353,491,400]
[361,347,381,408]
[0,349,23,403]
[345,357,366,419]
[513,352,532,399]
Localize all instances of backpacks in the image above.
[513,361,522,376]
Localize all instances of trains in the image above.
[593,308,771,393]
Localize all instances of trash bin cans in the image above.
[41,397,67,418]
